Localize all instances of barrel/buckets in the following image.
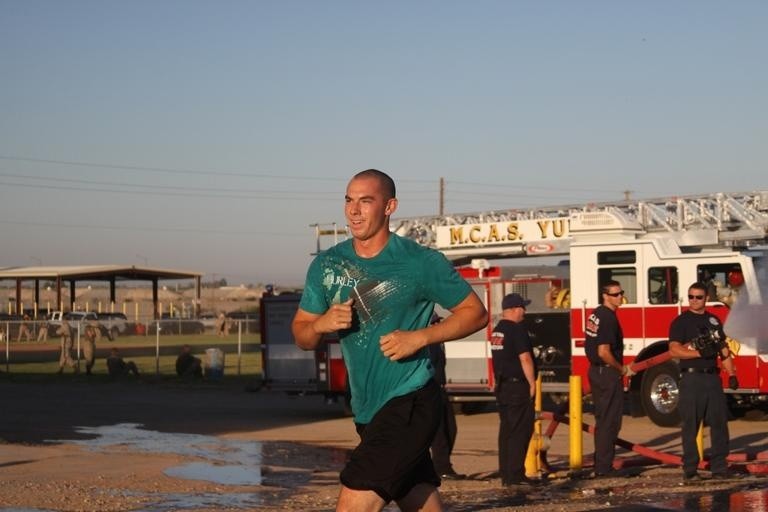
[206,347,225,379]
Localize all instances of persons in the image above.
[291,169,490,512]
[584,279,637,477]
[492,293,540,487]
[667,282,739,482]
[428,311,468,481]
[0,313,230,379]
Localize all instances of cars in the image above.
[47,311,130,339]
[149,318,206,335]
[199,311,218,329]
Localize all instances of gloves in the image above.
[729,375,739,390]
[698,345,717,359]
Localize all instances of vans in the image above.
[221,311,259,334]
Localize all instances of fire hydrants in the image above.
[525,412,551,481]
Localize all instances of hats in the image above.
[502,293,532,310]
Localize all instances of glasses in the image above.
[688,295,703,300]
[606,291,624,297]
[515,304,526,309]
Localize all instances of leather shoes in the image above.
[681,367,721,374]
[441,469,467,480]
[511,476,544,487]
[712,468,746,479]
[683,470,701,481]
[595,467,630,478]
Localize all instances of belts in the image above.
[501,377,519,383]
[592,363,613,368]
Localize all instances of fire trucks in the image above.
[326,193,767,416]
